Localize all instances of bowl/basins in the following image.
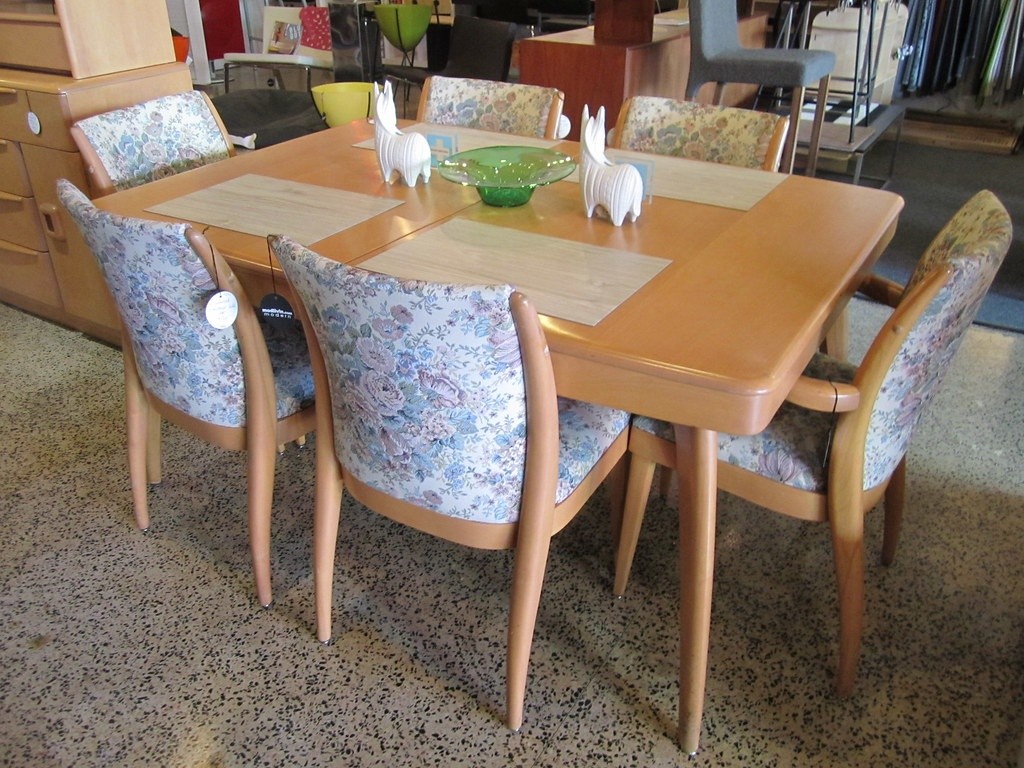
[438,146,575,207]
[376,6,430,53]
[311,83,384,128]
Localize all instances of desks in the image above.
[512,0,775,145]
[735,89,908,189]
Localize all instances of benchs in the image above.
[224,5,334,97]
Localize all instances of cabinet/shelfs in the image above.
[0,0,194,349]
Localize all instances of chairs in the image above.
[612,96,790,172]
[269,233,631,731]
[685,0,836,177]
[417,75,565,140]
[70,90,236,195]
[380,16,516,119]
[54,178,314,608]
[614,190,1012,698]
